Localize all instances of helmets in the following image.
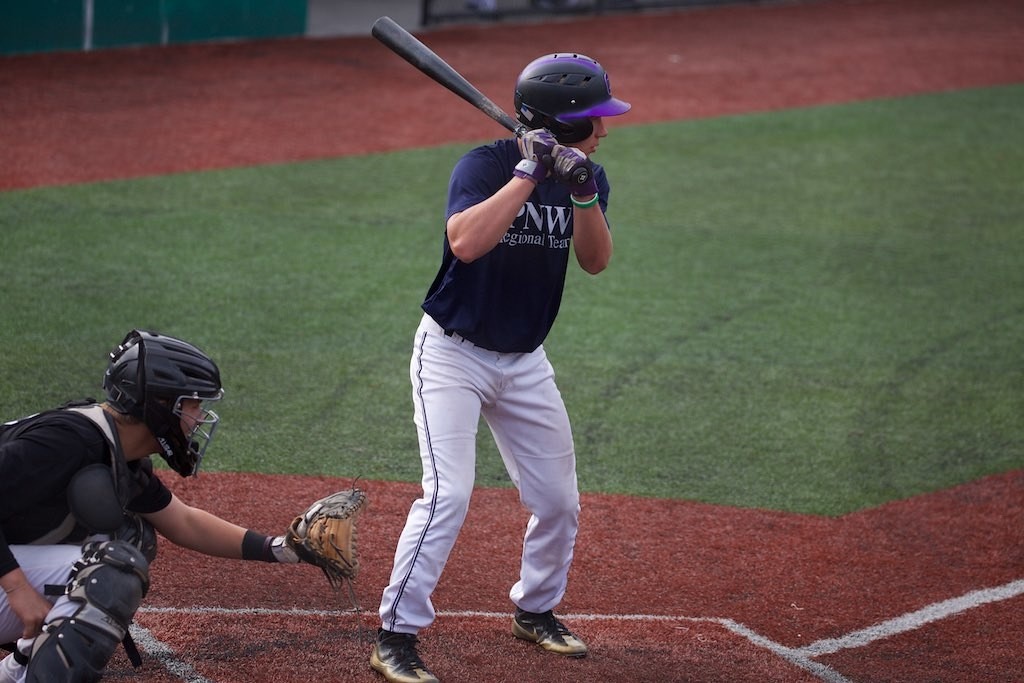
[103,329,225,477]
[514,53,631,143]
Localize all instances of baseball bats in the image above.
[371,15,590,186]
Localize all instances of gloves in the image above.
[513,126,558,187]
[542,145,599,196]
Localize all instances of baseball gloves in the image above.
[286,489,370,582]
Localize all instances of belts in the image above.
[443,328,479,351]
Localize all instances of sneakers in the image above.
[511,606,587,657]
[370,627,439,683]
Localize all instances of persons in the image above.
[0,329,365,683]
[371,52,632,683]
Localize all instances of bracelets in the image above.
[571,192,598,209]
